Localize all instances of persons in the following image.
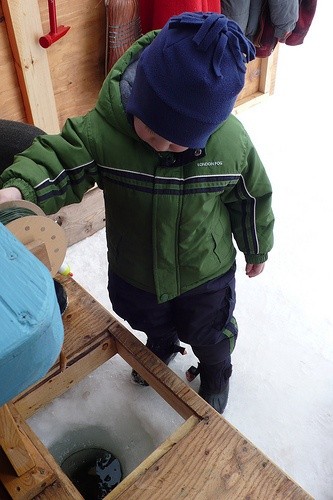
[1,10,273,416]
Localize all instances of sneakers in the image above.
[130,339,186,387]
[186,355,232,414]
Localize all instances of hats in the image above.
[127,12,256,149]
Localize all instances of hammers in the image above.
[39,0,71,48]
